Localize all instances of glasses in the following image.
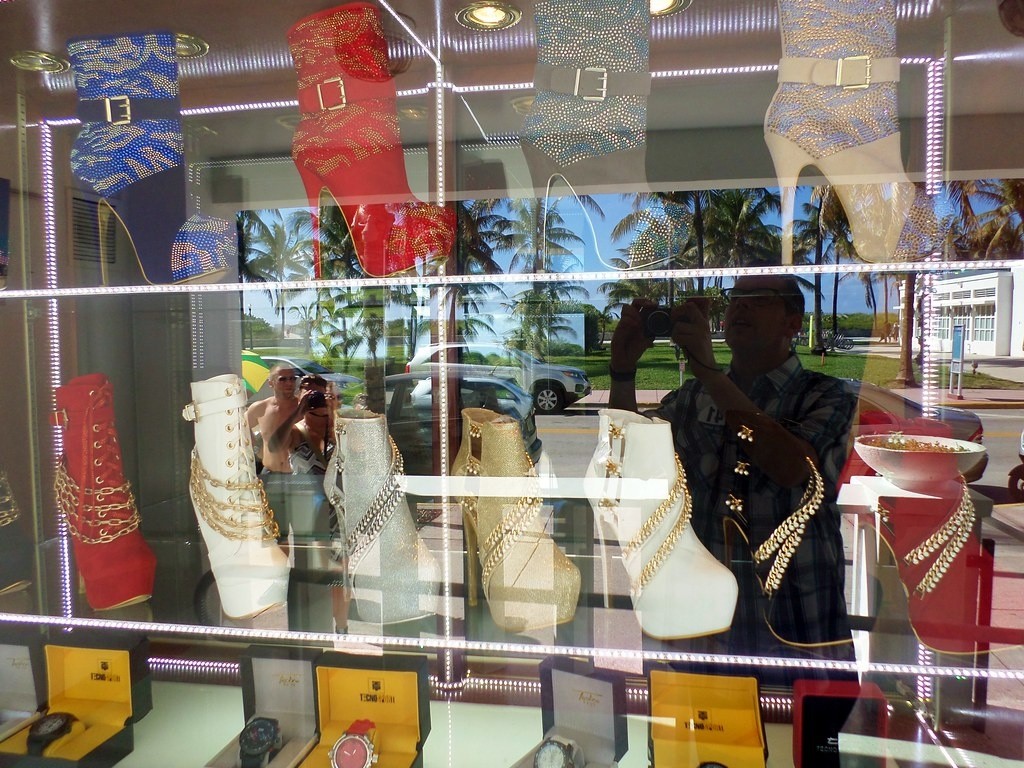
[298,375,328,391]
[720,288,792,307]
[277,375,300,382]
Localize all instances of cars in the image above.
[322,372,542,475]
[836,377,988,486]
[258,356,362,404]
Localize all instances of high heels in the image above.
[324,407,440,623]
[450,406,581,631]
[586,408,738,639]
[837,411,1023,655]
[708,419,854,646]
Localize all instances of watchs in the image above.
[237,716,283,768]
[27,711,79,756]
[534,735,586,768]
[327,719,379,768]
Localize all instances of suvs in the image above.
[405,341,590,414]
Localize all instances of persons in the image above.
[607,275,859,689]
[878,321,900,344]
[239,364,352,634]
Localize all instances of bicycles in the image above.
[794,329,856,351]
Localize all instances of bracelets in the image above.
[609,363,639,382]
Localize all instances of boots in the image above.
[287,3,456,280]
[66,30,238,286]
[520,0,692,273]
[764,0,957,264]
[182,374,290,620]
[49,373,157,612]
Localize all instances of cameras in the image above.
[642,307,689,338]
[307,390,333,408]
